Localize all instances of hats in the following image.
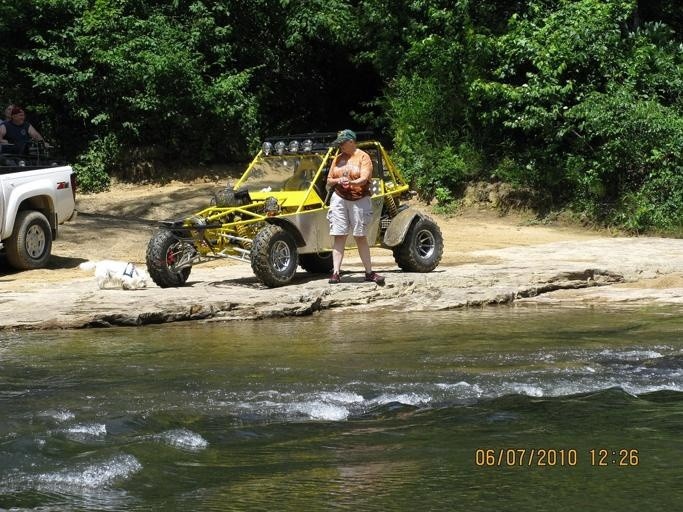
[331,128,356,145]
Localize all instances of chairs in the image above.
[283,158,322,213]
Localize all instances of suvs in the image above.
[146,130,444,288]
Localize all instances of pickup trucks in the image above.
[0,153,76,270]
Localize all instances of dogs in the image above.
[79,259,147,290]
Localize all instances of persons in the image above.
[0,102,50,171]
[326,128,386,284]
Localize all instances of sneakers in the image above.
[328,273,340,284]
[363,271,385,284]
